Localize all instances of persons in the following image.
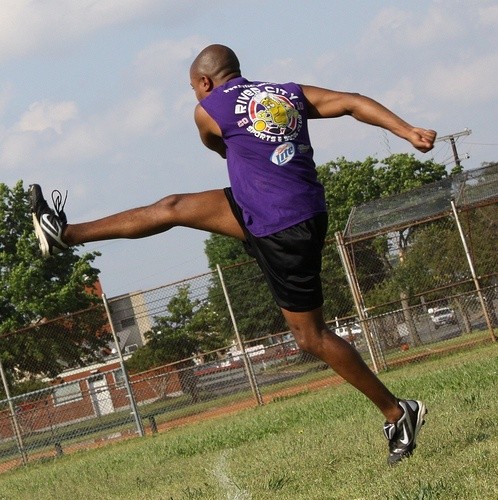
[28,44,437,466]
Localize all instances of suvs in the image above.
[431,306,457,330]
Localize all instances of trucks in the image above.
[334,327,363,340]
[216,344,266,370]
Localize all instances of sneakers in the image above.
[31,183,72,261]
[383,399,429,466]
